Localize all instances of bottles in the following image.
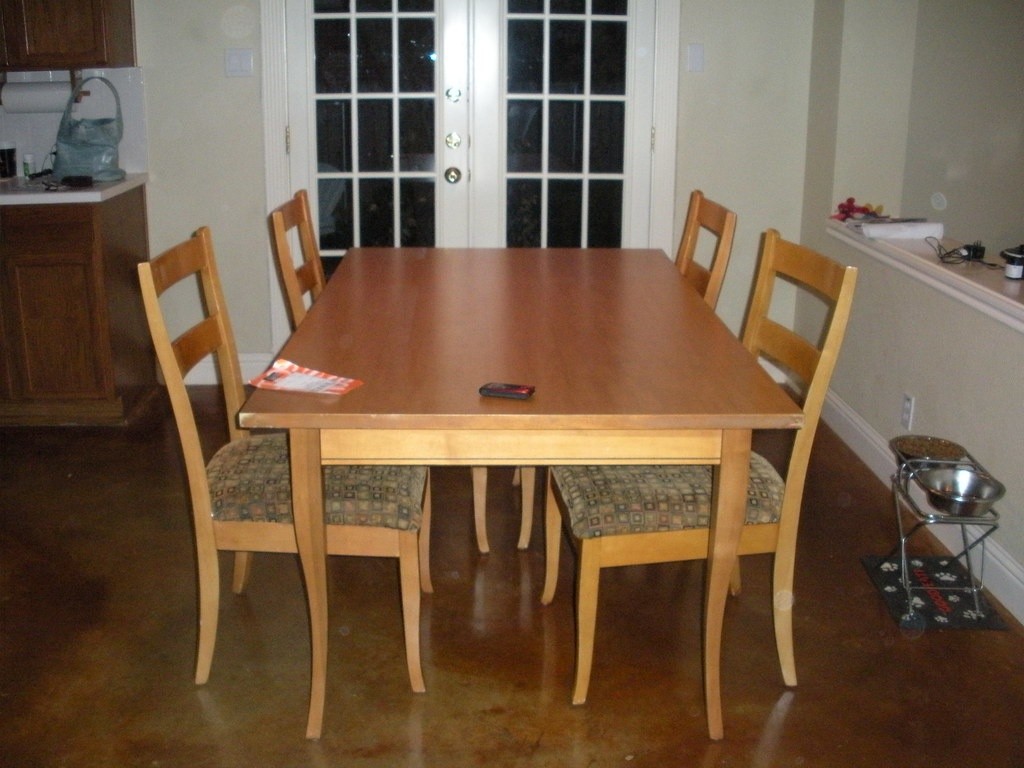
[23,154,36,179]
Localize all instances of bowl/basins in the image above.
[888,435,967,473]
[919,470,1006,517]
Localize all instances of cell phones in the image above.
[479,382,535,399]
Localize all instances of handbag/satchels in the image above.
[51,78,127,181]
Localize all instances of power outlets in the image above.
[901,394,915,432]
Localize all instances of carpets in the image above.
[859,555,1007,631]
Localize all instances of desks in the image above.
[238,248,805,741]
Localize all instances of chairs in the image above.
[511,190,736,549]
[138,225,433,693]
[541,228,857,705]
[271,189,490,553]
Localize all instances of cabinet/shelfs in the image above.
[0,0,134,71]
[0,184,158,428]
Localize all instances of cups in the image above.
[0,142,16,180]
